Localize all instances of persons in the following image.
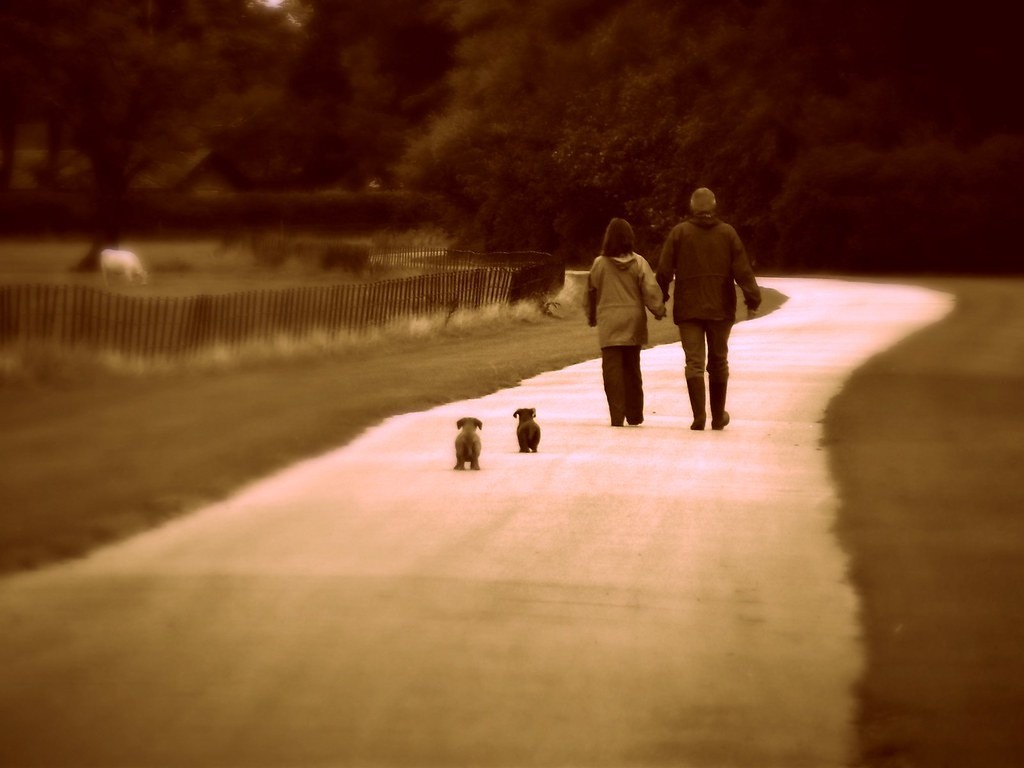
[580,219,665,427]
[653,189,763,430]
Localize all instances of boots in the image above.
[686,376,706,430]
[708,381,729,430]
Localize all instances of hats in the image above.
[690,188,716,211]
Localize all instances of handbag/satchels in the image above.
[586,275,597,326]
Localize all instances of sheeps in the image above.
[101,249,148,286]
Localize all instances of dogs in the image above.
[514,409,540,453]
[454,418,483,470]
